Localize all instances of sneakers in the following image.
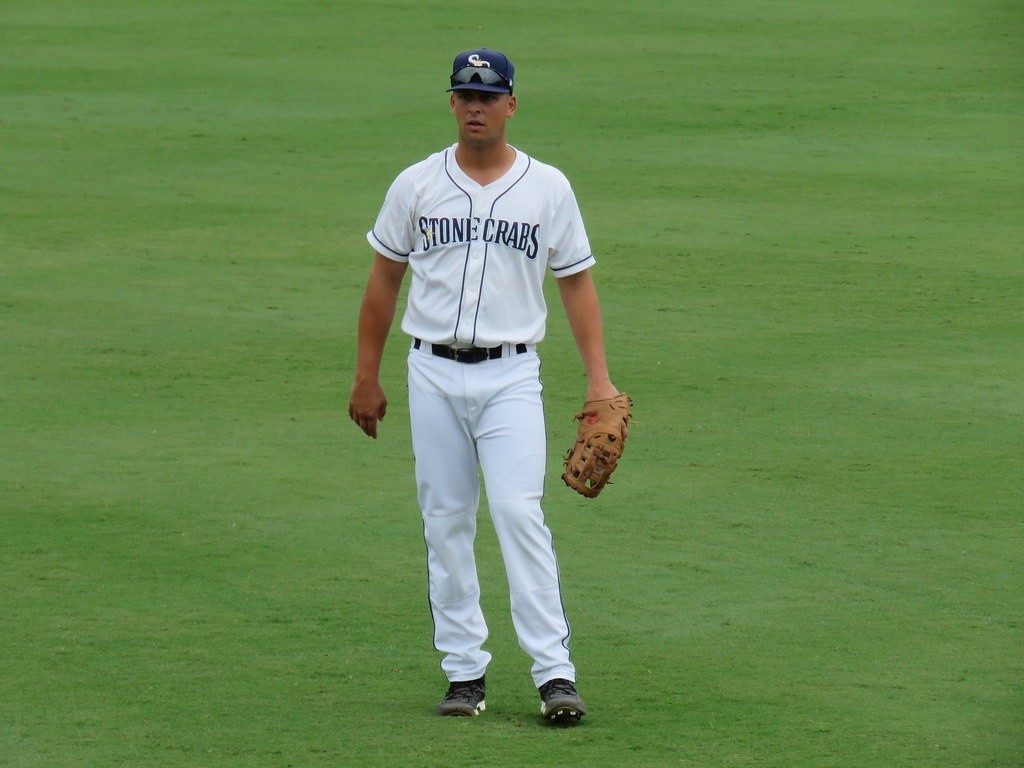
[539,678,586,721]
[438,674,486,717]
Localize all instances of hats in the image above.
[446,47,515,93]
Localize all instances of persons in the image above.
[345,47,636,726]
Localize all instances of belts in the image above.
[414,338,527,363]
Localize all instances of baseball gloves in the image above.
[561,391,631,499]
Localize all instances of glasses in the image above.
[450,66,512,96]
